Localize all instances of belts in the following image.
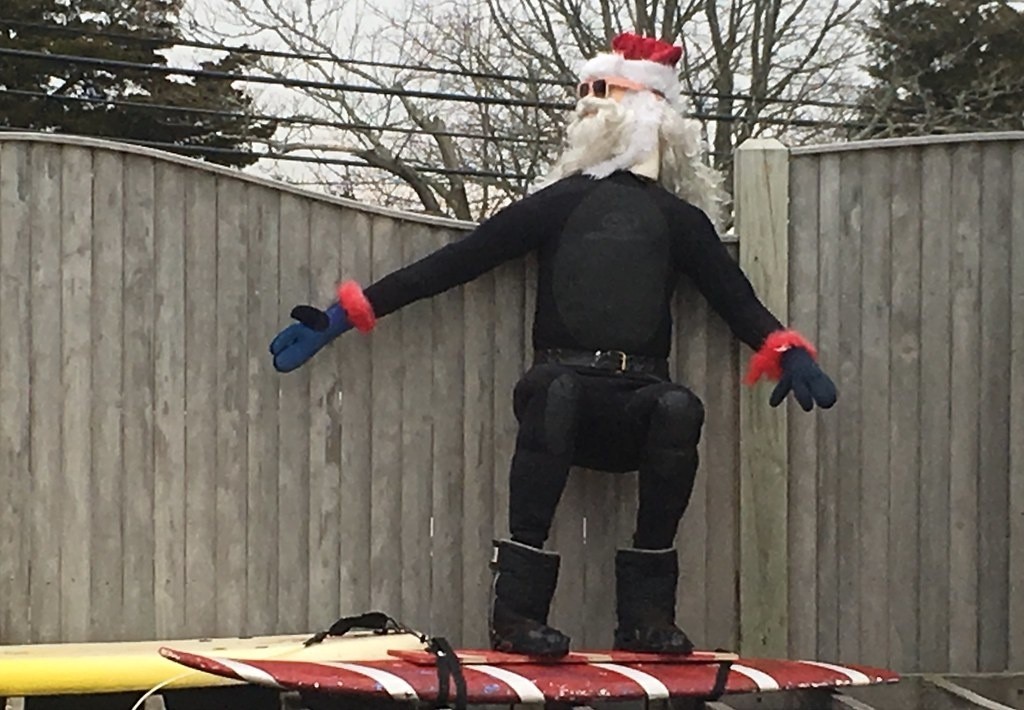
[540,348,669,375]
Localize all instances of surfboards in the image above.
[0,629,431,696]
[158,646,902,703]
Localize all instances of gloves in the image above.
[749,329,838,412]
[270,283,378,373]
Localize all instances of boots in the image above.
[489,538,570,657]
[614,546,694,654]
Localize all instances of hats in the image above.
[579,32,683,107]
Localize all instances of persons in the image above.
[267,34,837,662]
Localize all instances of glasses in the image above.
[577,79,673,103]
